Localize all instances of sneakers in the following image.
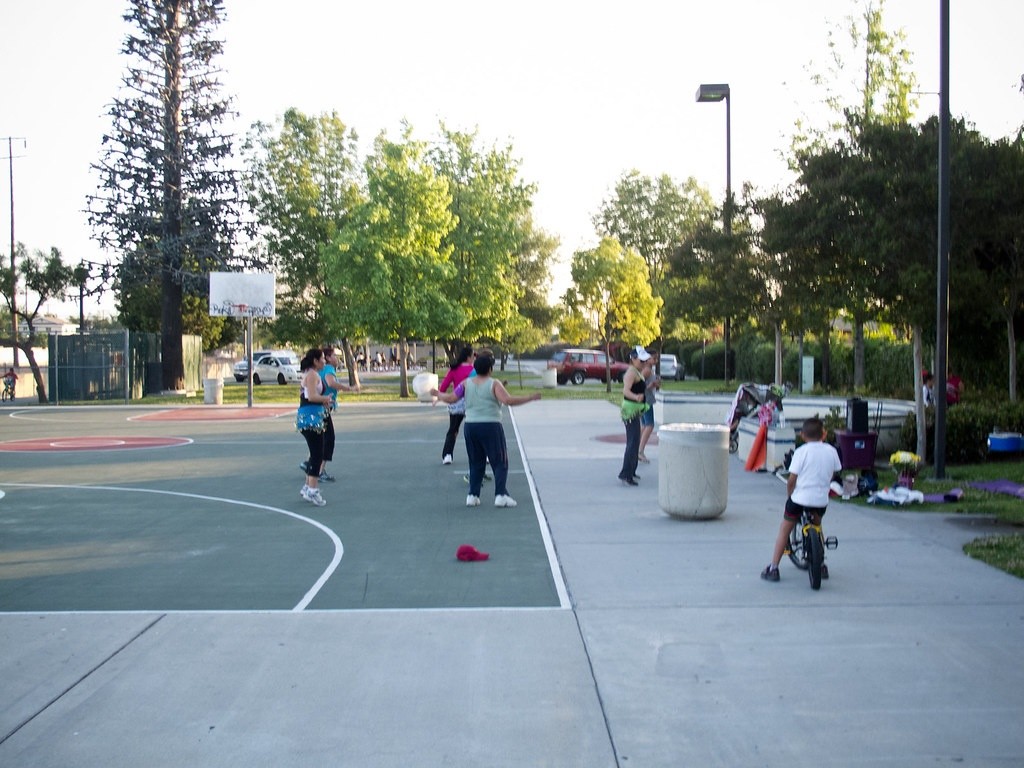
[494,494,517,507]
[442,454,452,465]
[303,489,326,507]
[319,469,335,483]
[300,484,309,496]
[300,461,309,471]
[466,495,481,507]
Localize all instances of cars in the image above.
[251,354,306,387]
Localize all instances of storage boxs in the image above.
[832,431,877,469]
[989,431,1023,452]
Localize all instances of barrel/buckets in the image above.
[543,368,557,388]
[658,423,730,519]
[417,374,438,401]
[203,379,224,404]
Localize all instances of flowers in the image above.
[890,450,923,476]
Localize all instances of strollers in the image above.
[726,381,795,454]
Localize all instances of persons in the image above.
[407,352,413,369]
[433,345,509,485]
[923,374,934,407]
[430,352,542,507]
[947,368,964,405]
[375,351,396,371]
[355,351,364,371]
[301,348,360,481]
[760,418,841,581]
[0,368,18,399]
[639,351,661,464]
[618,344,650,485]
[296,348,331,506]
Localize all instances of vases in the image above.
[897,472,915,491]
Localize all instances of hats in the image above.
[646,347,658,357]
[630,345,651,362]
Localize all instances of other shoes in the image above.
[821,564,829,580]
[634,472,641,479]
[482,472,492,482]
[760,565,780,581]
[463,474,483,488]
[618,475,638,486]
[636,455,650,464]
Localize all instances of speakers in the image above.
[846,400,869,433]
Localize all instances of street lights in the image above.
[693,83,733,391]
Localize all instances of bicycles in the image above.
[0,376,18,402]
[783,510,838,591]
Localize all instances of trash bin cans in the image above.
[659,422,729,519]
[203,378,223,405]
[413,372,439,403]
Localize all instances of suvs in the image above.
[546,348,629,385]
[653,353,686,382]
[233,349,296,382]
